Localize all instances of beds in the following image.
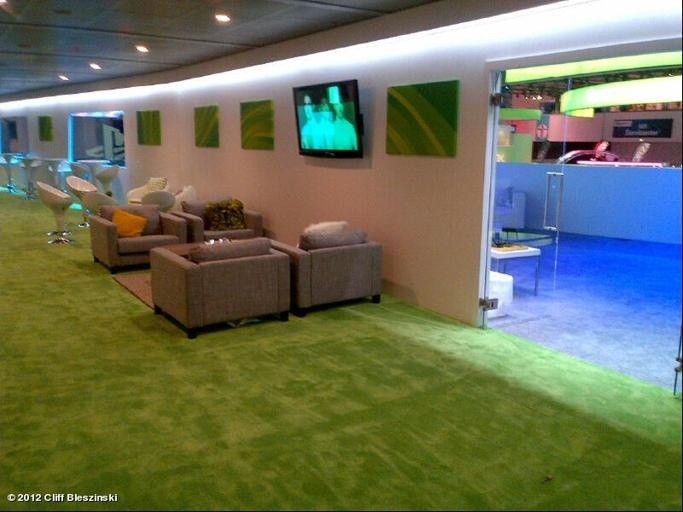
[491,228,553,297]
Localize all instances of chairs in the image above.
[0,152,197,246]
[88,201,383,340]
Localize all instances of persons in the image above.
[302,87,358,150]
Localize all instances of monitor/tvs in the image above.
[292,78,363,159]
